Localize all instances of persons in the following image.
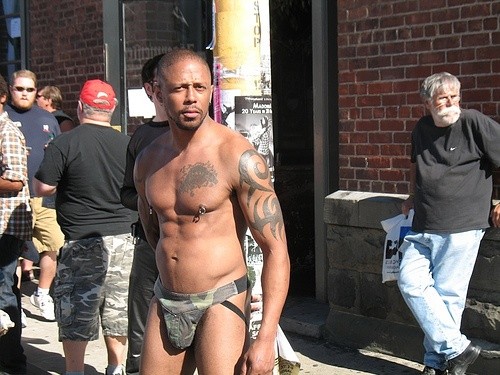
[133,47,290,375]
[3,69,65,320]
[254,116,271,165]
[397,72,500,375]
[33,80,139,375]
[121,54,170,375]
[35,85,75,132]
[0,74,33,375]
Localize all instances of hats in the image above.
[81,80,117,111]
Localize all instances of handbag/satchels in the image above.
[381,209,414,283]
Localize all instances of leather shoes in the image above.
[421,366,448,375]
[446,343,481,375]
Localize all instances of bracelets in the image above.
[410,192,415,196]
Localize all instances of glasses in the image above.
[36,94,45,98]
[13,85,36,93]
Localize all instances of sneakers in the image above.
[30,292,56,321]
[20,309,28,328]
